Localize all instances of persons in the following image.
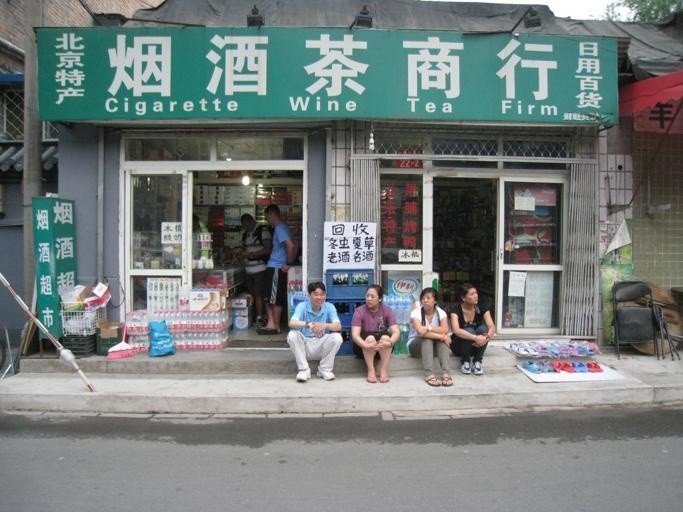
[450,284,495,376]
[287,282,343,381]
[256,205,297,335]
[230,214,271,328]
[193,214,211,258]
[352,285,400,383]
[406,288,453,386]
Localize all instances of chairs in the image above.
[612,280,660,360]
[653,304,681,361]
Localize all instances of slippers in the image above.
[530,339,599,356]
[510,341,537,356]
[571,361,587,372]
[585,361,602,372]
[560,362,574,372]
[522,360,540,373]
[256,327,277,335]
[550,360,561,372]
[536,358,552,372]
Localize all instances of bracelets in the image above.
[440,333,444,341]
[484,333,491,339]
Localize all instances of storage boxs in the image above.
[325,327,353,356]
[60,302,107,336]
[95,321,123,356]
[231,296,250,309]
[190,288,221,311]
[232,305,254,331]
[325,269,374,297]
[325,300,366,326]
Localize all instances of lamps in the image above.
[226,158,232,161]
[369,133,375,150]
[242,176,250,186]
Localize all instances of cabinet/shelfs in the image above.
[193,178,303,251]
[196,232,214,269]
[192,266,244,298]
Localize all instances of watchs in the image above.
[249,252,254,259]
[286,263,292,265]
[306,322,309,328]
[428,326,432,331]
[389,340,392,346]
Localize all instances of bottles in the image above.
[382,294,412,354]
[333,273,347,285]
[289,280,308,309]
[127,310,228,351]
[353,272,368,283]
[335,303,349,313]
[179,287,190,311]
[344,333,350,341]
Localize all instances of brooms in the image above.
[107,325,133,359]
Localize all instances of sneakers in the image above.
[315,365,334,380]
[461,362,470,374]
[296,368,310,381]
[473,361,483,375]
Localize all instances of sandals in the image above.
[379,371,389,383]
[365,370,376,383]
[425,373,441,386]
[441,372,452,386]
